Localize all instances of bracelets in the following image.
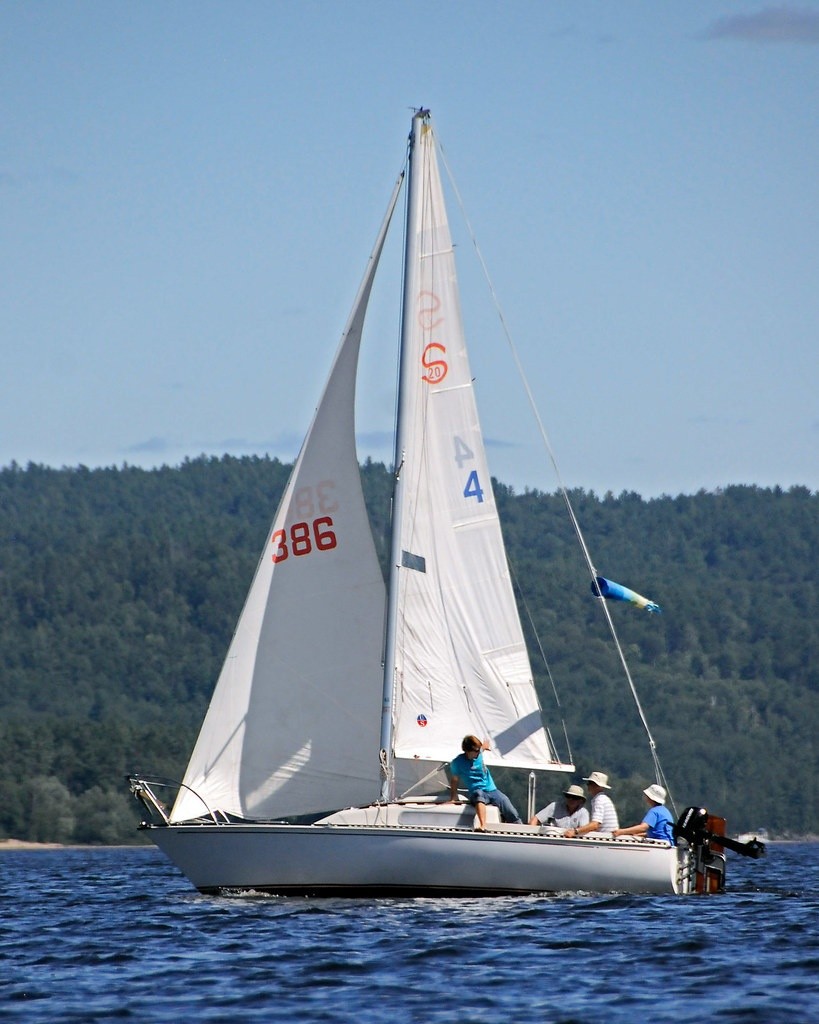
[574,828,578,835]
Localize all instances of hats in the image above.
[582,771,612,789]
[563,784,587,801]
[643,784,666,805]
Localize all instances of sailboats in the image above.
[125,105,765,893]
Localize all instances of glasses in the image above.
[470,748,479,752]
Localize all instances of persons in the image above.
[450,735,523,832]
[565,772,619,838]
[611,784,674,846]
[529,785,589,828]
